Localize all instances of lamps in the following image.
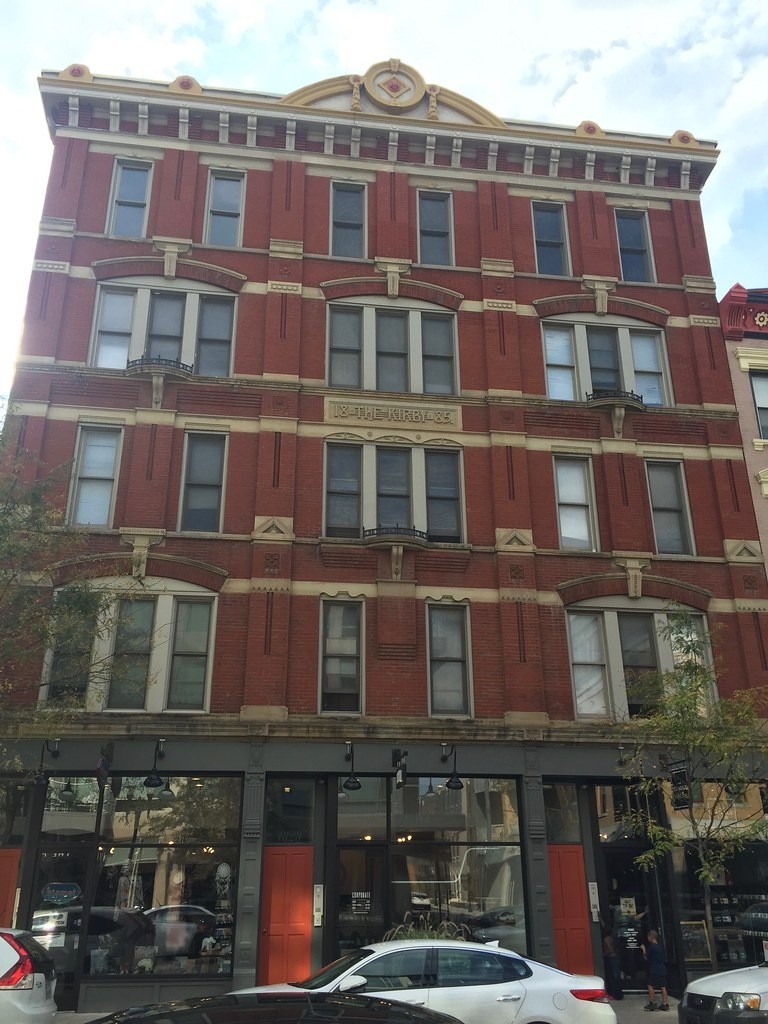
[35,739,59,786]
[441,745,464,790]
[143,740,165,787]
[343,743,362,791]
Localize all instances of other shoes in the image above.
[658,1003,669,1009]
[644,1003,658,1011]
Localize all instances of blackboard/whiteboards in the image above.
[681,919,712,962]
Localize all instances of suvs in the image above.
[678,961,768,1024]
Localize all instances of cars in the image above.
[83,939,618,1024]
[0,928,58,1024]
[33,904,229,975]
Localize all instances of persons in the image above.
[641,931,670,1011]
[604,935,623,999]
[598,894,650,978]
